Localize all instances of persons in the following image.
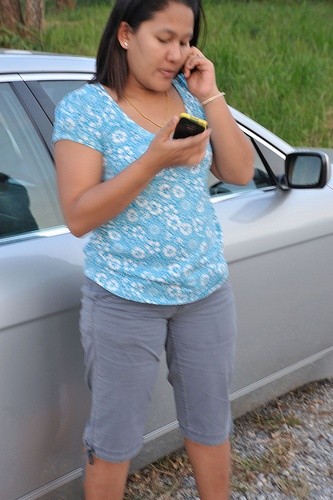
[50,0,255,500]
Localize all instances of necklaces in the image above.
[115,91,172,130]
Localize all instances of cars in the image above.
[0,49,333,500]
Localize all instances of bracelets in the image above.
[197,93,224,106]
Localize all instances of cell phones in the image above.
[172,112,208,139]
